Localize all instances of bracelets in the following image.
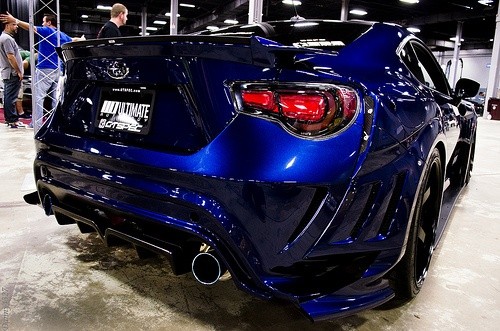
[15,18,19,23]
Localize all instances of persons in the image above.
[15,50,38,119]
[0,10,72,133]
[475,91,485,105]
[0,22,29,129]
[97,3,128,39]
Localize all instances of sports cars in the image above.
[24,19,481,323]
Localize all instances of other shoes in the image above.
[18,113,32,118]
[9,122,25,129]
